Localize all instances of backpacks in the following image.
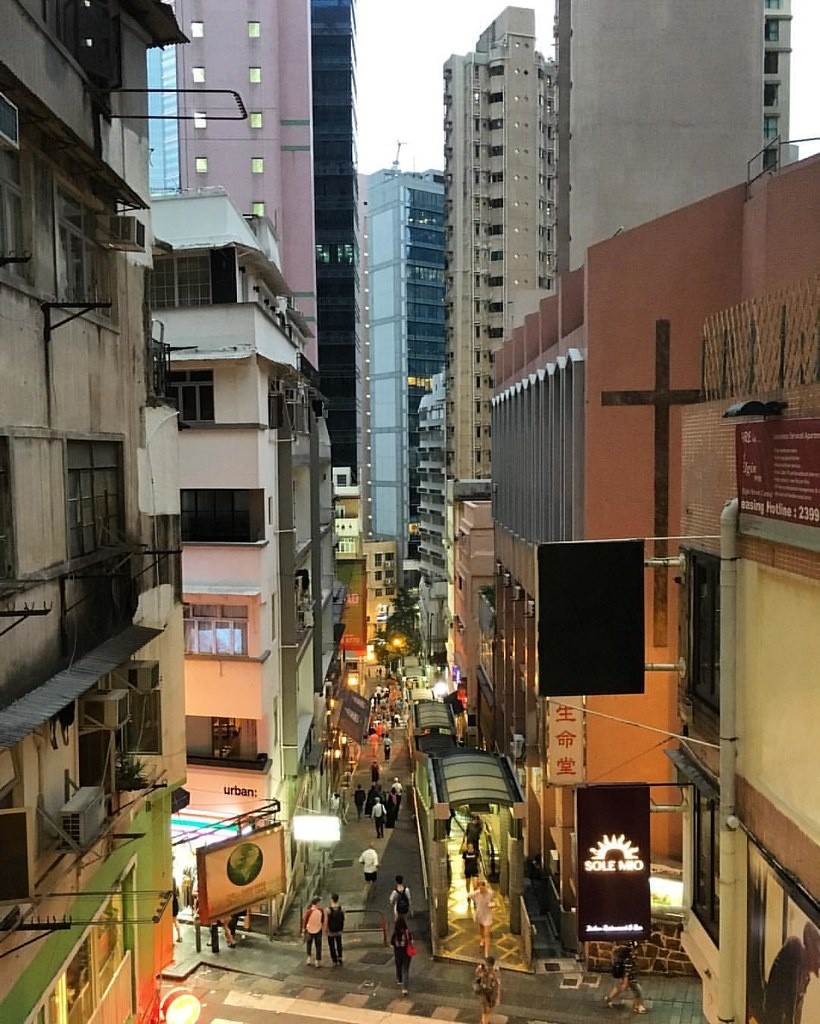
[394,887,409,914]
[613,955,633,978]
[328,906,344,932]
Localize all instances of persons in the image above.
[301,896,325,967]
[330,674,413,839]
[468,881,493,958]
[207,913,240,948]
[447,855,452,890]
[603,942,647,1014]
[389,875,413,924]
[389,917,414,995]
[172,895,183,942]
[324,893,347,967]
[359,842,382,901]
[463,816,482,909]
[474,956,503,1024]
[445,809,456,837]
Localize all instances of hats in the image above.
[628,940,638,949]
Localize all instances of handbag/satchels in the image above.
[472,977,483,991]
[406,946,416,957]
[459,842,468,854]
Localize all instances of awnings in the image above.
[338,703,365,744]
[240,243,315,339]
[345,688,371,717]
[0,625,165,750]
[120,1,191,50]
[0,58,152,214]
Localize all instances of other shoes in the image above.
[633,1005,647,1014]
[339,960,343,964]
[306,958,310,964]
[207,942,213,946]
[605,996,615,1007]
[228,941,235,947]
[176,938,182,942]
[333,959,338,962]
[402,989,407,994]
[398,981,403,985]
[315,960,319,967]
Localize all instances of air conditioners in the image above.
[111,215,147,250]
[0,91,20,151]
[127,659,161,691]
[83,687,130,729]
[63,786,105,846]
